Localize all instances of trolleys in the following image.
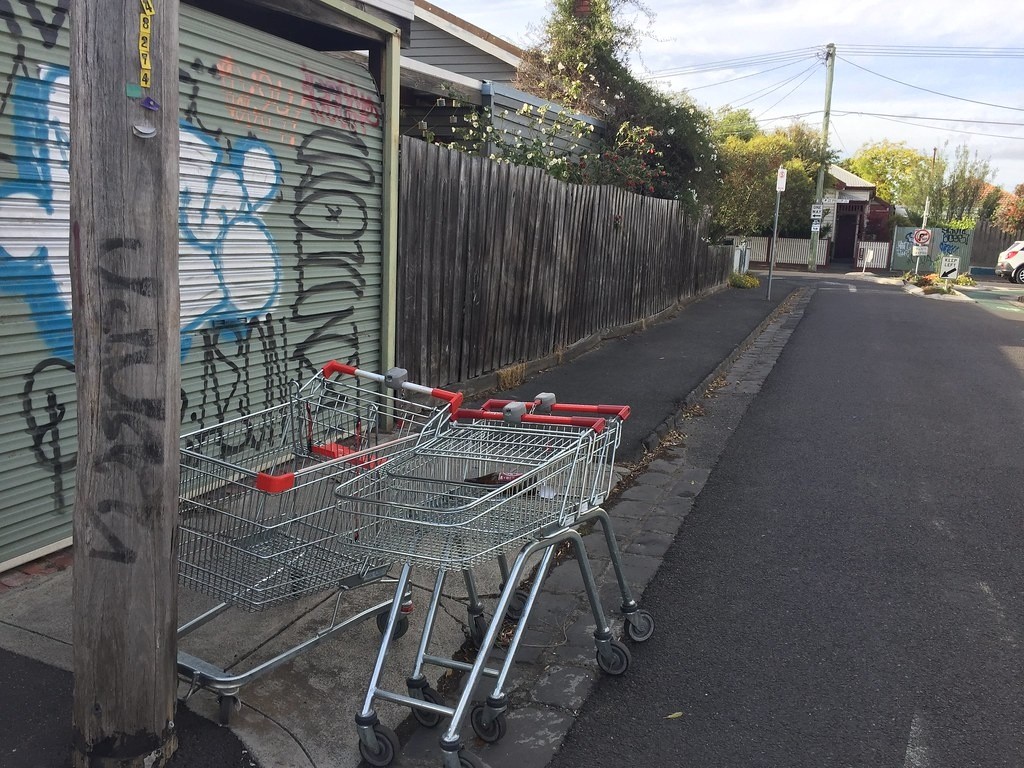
[175,359,462,725]
[331,392,657,768]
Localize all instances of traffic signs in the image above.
[811,204,823,220]
[939,256,960,279]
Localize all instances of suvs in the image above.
[995,239,1024,285]
